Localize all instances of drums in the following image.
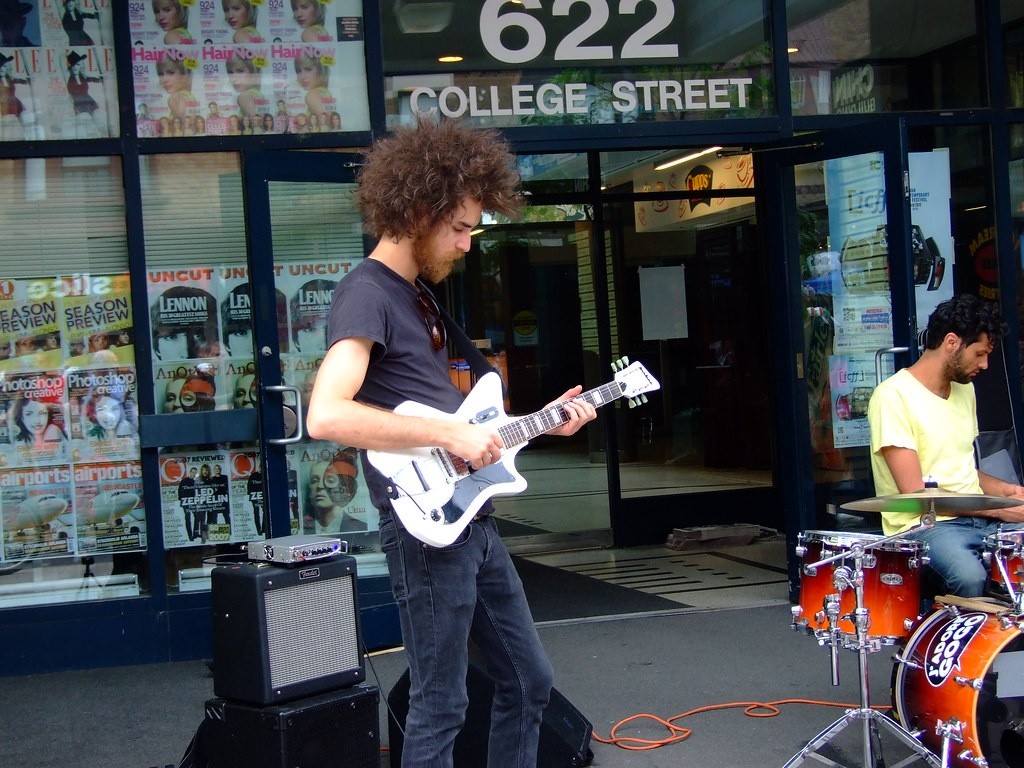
[890,597,1024,768]
[794,529,926,651]
[977,528,1024,602]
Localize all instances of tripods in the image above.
[781,501,938,768]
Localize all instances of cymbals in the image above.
[838,486,1024,515]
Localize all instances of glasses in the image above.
[417,292,448,351]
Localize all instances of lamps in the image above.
[787,42,803,54]
[437,42,464,62]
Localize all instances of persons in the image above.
[866,296,1024,598]
[306,108,598,767]
[1,0,342,137]
[0,279,368,546]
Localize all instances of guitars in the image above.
[362,353,662,553]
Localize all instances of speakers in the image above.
[388,663,594,768]
[203,685,381,768]
[211,553,367,705]
